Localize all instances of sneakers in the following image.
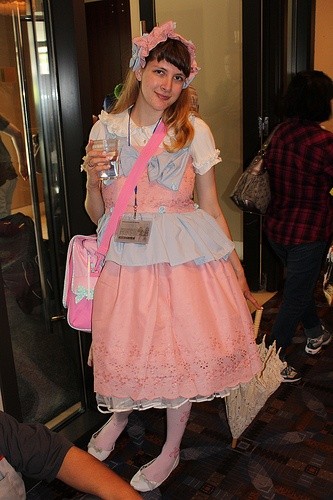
[305,329,331,355]
[280,364,302,382]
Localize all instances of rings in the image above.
[87,164,92,167]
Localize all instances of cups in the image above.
[90,139,119,177]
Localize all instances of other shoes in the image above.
[87,414,127,461]
[130,454,179,492]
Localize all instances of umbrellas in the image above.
[223,309,287,447]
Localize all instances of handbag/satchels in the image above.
[61,234,105,333]
[228,121,288,214]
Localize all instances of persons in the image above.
[83,21,264,492]
[0,410,144,500]
[261,70,333,381]
[0,114,29,219]
